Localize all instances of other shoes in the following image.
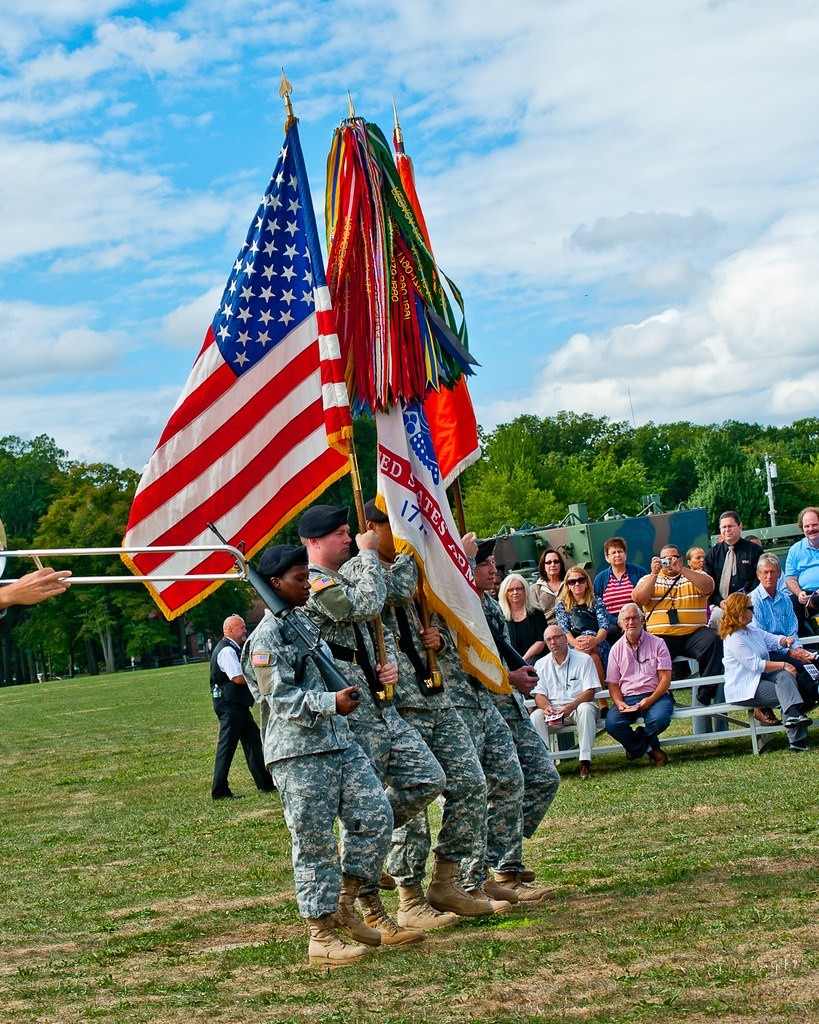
[228,793,246,799]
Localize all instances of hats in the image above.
[365,499,389,524]
[475,539,497,564]
[300,505,351,539]
[257,542,309,584]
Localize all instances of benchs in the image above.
[525,635,819,767]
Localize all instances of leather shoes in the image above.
[789,742,813,752]
[646,736,667,767]
[579,762,594,781]
[784,716,813,727]
[754,708,780,725]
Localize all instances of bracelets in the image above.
[783,661,787,670]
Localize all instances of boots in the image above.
[377,869,396,890]
[517,869,535,882]
[327,875,381,947]
[357,891,424,946]
[305,917,371,967]
[425,855,512,915]
[396,883,459,932]
[494,867,554,902]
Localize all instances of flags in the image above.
[121,115,354,620]
[373,127,513,692]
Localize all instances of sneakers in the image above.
[482,877,518,904]
[463,885,512,914]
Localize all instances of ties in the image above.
[719,546,737,599]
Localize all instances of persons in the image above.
[530,625,602,781]
[182,645,189,664]
[0,567,72,621]
[720,591,812,751]
[494,507,819,726]
[206,639,216,661]
[604,604,673,767]
[240,497,560,966]
[210,616,277,799]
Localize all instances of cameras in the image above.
[660,559,670,567]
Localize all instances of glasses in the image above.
[567,576,587,586]
[546,633,565,644]
[545,560,560,565]
[607,550,623,556]
[747,606,754,612]
[507,586,525,592]
[660,556,682,561]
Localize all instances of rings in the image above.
[390,675,392,680]
